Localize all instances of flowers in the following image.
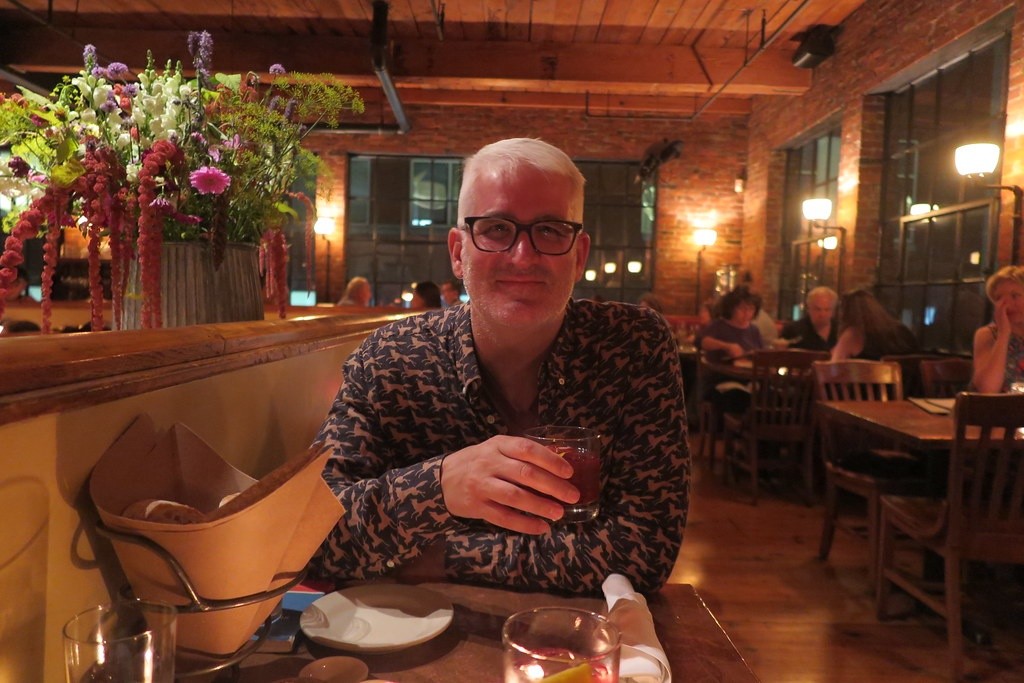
[0,27,364,332]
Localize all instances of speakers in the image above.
[792,30,834,69]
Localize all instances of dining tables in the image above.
[77,571,762,683]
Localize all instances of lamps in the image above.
[955,142,1022,265]
[694,230,717,316]
[803,199,846,300]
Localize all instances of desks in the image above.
[702,356,812,493]
[818,398,1024,646]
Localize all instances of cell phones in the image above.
[250,590,326,653]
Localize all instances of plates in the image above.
[300,586,455,655]
[299,656,368,682]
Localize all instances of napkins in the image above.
[601,573,672,683]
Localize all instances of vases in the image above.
[112,241,265,333]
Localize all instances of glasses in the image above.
[464,215,582,256]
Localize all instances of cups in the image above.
[502,607,622,683]
[524,425,601,523]
[62,595,178,683]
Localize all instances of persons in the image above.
[639,293,665,313]
[411,281,464,310]
[691,286,778,464]
[337,277,372,306]
[306,137,692,593]
[774,286,838,352]
[830,276,924,402]
[966,266,1024,393]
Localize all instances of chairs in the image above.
[690,334,1024,683]
